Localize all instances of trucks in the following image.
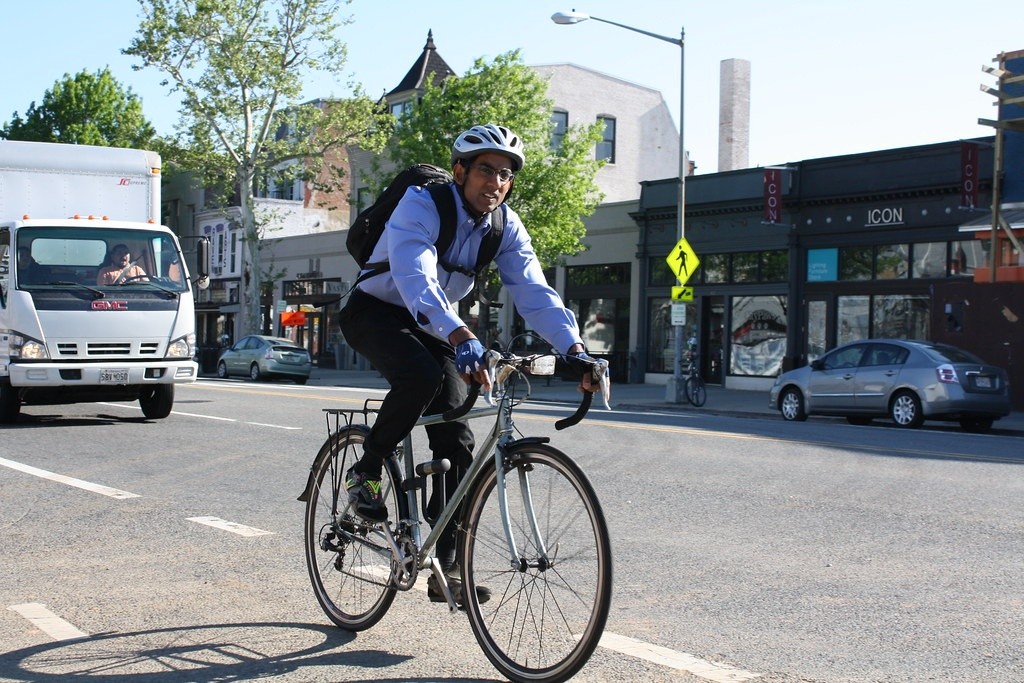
[0,137,212,425]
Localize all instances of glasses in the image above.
[470,164,514,181]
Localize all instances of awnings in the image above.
[194,302,241,314]
[263,293,341,311]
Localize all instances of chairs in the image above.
[98,251,113,272]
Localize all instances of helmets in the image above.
[451,124,525,169]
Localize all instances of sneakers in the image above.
[343,462,388,522]
[427,574,492,604]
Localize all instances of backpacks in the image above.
[346,164,503,277]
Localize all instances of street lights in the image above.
[549,9,687,402]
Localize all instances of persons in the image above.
[97,244,150,286]
[17,246,49,285]
[336,123,611,604]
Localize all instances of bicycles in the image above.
[680,349,708,407]
[291,326,615,683]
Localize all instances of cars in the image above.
[217,335,313,385]
[768,338,1013,434]
